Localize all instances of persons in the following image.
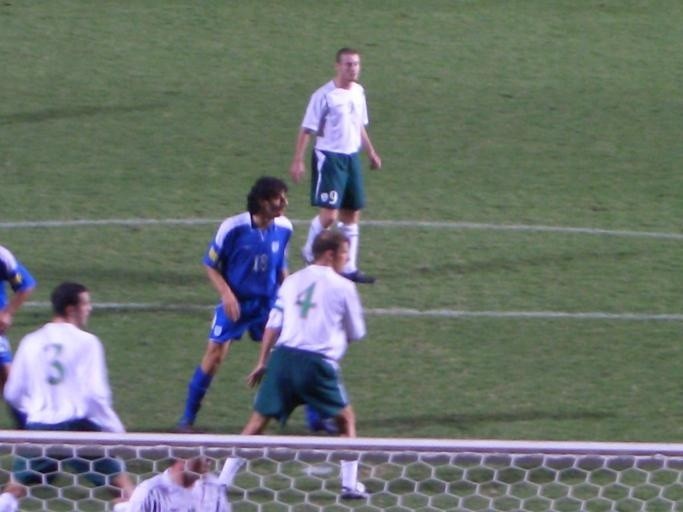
[287,45,383,285]
[174,174,337,433]
[216,227,371,499]
[0,280,139,512]
[110,424,231,512]
[0,243,36,430]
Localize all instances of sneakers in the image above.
[340,269,375,283]
[341,482,365,499]
